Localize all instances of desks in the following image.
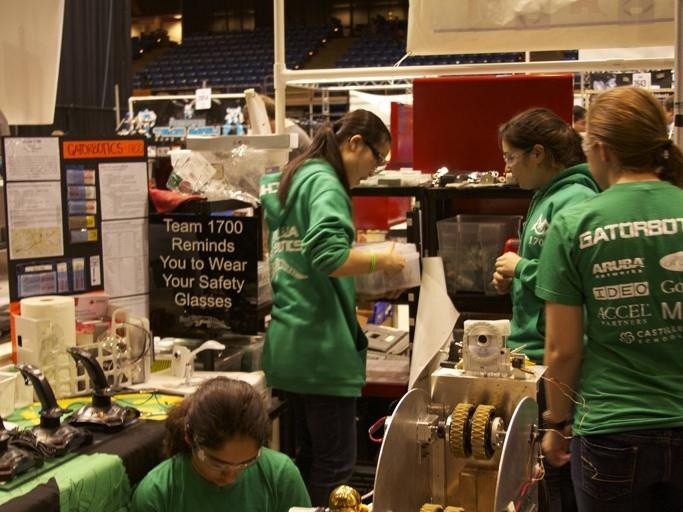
[357,352,409,495]
[0,337,292,511]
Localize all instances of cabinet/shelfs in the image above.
[273,0,683,319]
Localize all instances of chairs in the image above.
[130,29,508,94]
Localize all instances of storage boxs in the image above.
[351,239,422,296]
[436,211,523,297]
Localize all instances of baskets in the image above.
[12,304,134,401]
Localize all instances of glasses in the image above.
[198,451,262,473]
[364,140,387,166]
[502,149,530,163]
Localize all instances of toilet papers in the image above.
[407,256,460,394]
[19,296,77,398]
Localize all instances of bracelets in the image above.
[369,252,377,274]
[545,418,573,430]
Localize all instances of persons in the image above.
[260,108,406,509]
[490,108,601,510]
[243,95,310,161]
[573,105,587,133]
[130,376,312,511]
[540,85,682,512]
[663,94,674,135]
